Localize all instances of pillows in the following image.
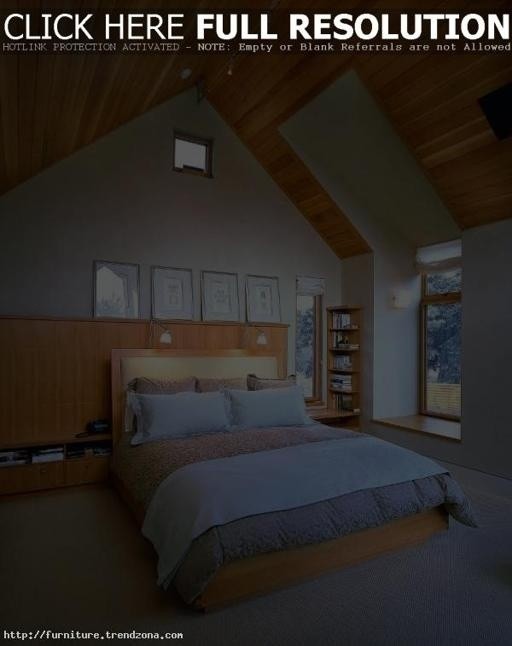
[126,372,317,448]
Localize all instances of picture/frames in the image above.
[91,258,283,326]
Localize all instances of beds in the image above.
[110,347,454,617]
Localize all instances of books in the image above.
[330,310,361,413]
[28,445,65,466]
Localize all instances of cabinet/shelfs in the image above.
[326,302,364,410]
[1,434,111,496]
